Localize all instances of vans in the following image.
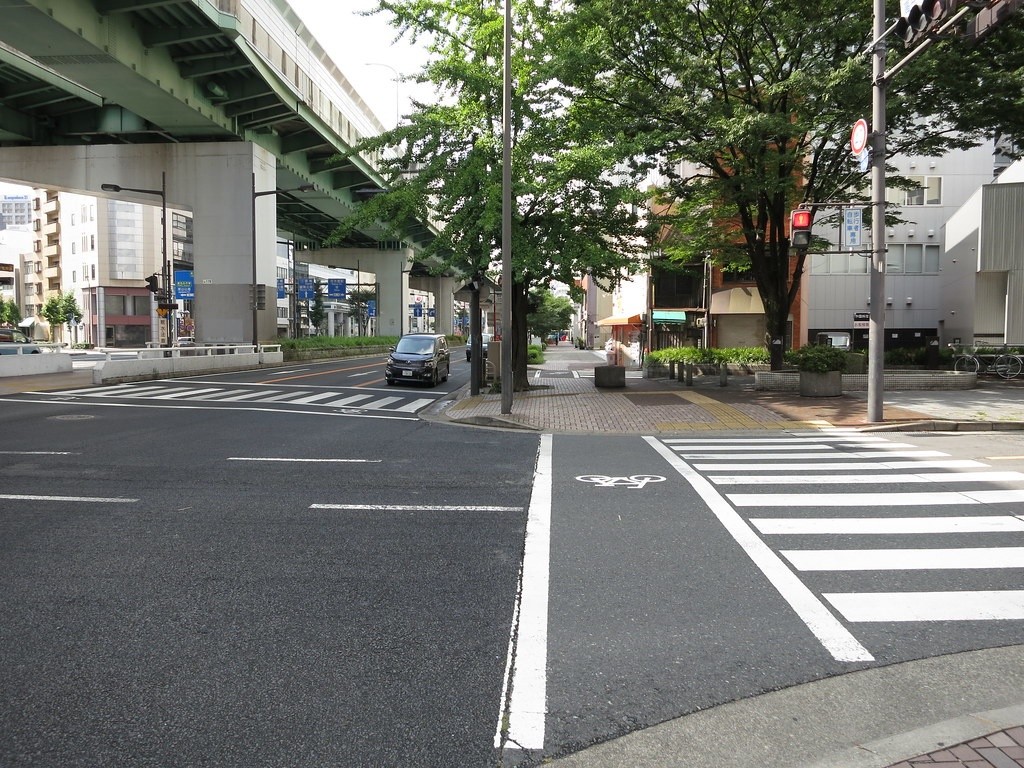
[0,327,42,355]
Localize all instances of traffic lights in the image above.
[893,0,956,50]
[792,210,812,228]
[145,276,158,292]
[792,230,811,248]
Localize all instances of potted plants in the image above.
[575,337,586,349]
[796,341,843,398]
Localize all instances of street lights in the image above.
[327,259,361,338]
[399,261,428,338]
[101,170,172,357]
[250,171,319,355]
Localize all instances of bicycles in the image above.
[953,340,1024,380]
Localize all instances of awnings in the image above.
[652,311,686,325]
[18,317,34,327]
[594,310,643,325]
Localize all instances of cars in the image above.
[466,333,494,361]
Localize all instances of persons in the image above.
[555,334,558,345]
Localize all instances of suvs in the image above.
[384,332,451,387]
